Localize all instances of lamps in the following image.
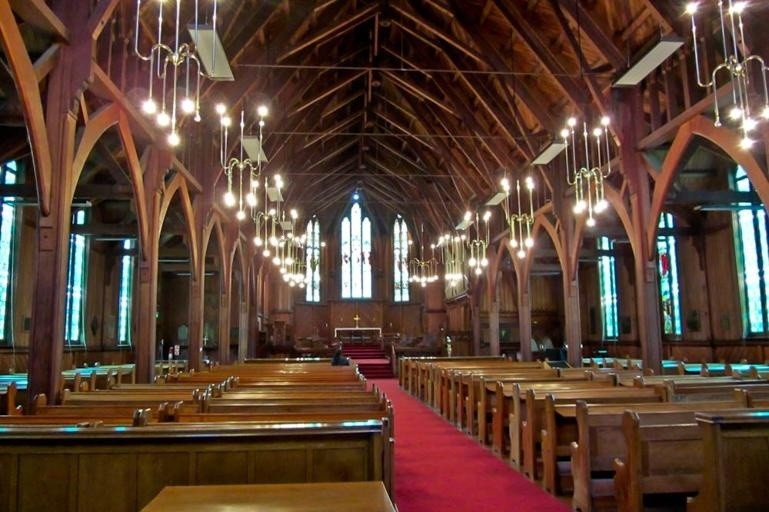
[682,0,769,153]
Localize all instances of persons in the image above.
[331,349,349,365]
